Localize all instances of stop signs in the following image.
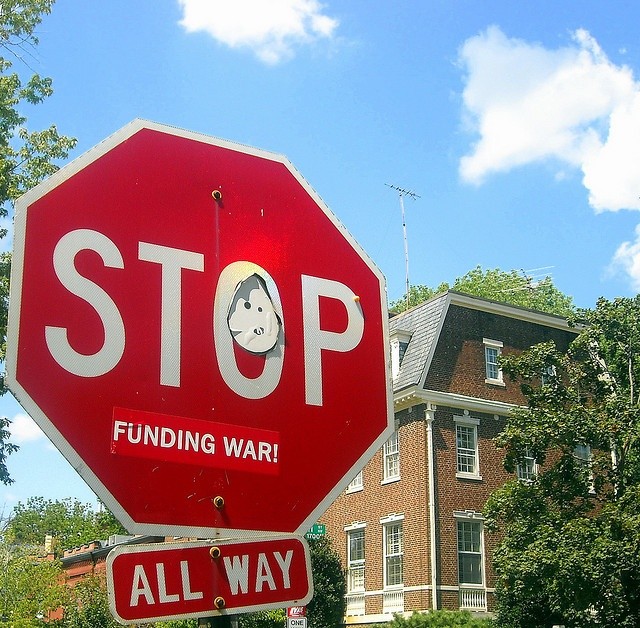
[5,117,397,543]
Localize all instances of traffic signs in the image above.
[106,534,315,626]
[305,525,325,539]
